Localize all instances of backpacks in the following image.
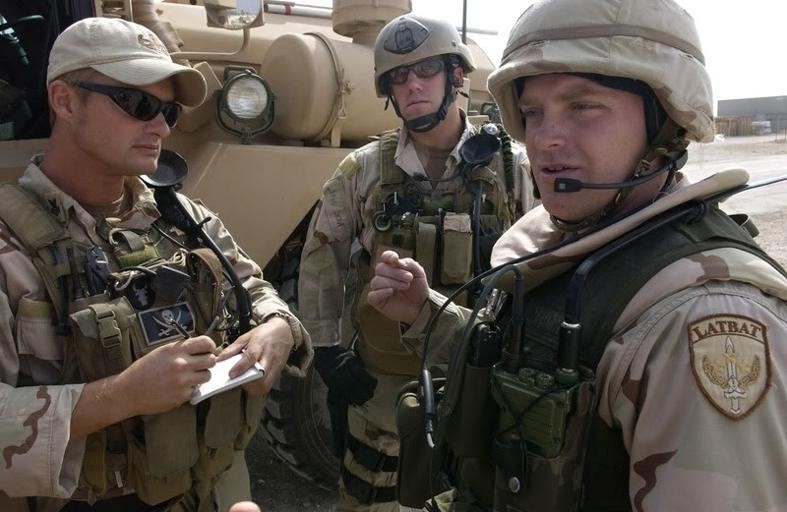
[397,203,787,511]
[355,130,512,370]
[0,183,264,505]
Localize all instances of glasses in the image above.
[383,59,449,84]
[66,81,183,127]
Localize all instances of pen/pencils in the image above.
[168,318,190,339]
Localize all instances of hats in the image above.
[45,18,208,109]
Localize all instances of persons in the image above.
[298,11,542,512]
[0,17,314,512]
[367,0,787,512]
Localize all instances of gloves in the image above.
[320,347,378,404]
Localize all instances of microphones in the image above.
[553,149,688,193]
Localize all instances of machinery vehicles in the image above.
[1,0,495,494]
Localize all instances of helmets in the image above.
[373,13,479,98]
[487,0,715,141]
